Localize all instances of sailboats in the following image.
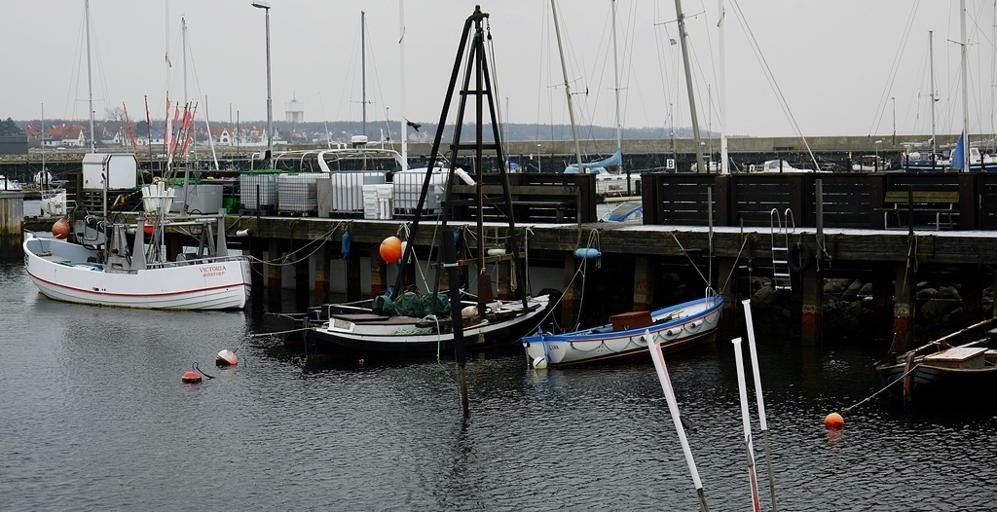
[539,0,996,197]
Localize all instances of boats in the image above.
[877,317,996,410]
[307,294,552,358]
[23,173,253,311]
[522,296,724,369]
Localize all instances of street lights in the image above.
[251,2,273,169]
